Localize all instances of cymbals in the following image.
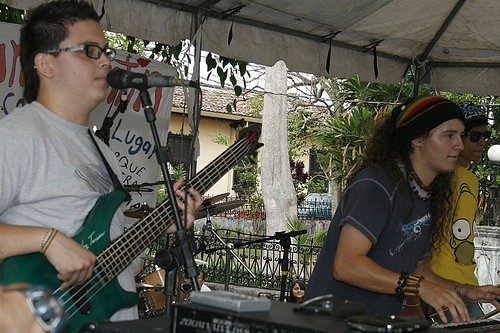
[196,192,230,210]
[140,255,209,266]
[194,200,248,220]
[124,208,156,219]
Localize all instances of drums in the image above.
[135,263,194,320]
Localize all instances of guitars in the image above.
[0,118,265,333]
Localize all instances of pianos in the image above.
[428,318,500,333]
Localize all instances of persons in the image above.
[285,279,305,304]
[416,100,487,321]
[197,269,211,293]
[0,0,202,323]
[300,96,500,323]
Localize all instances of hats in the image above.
[456,101,487,122]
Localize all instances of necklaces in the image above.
[407,167,432,200]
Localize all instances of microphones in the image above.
[106,69,198,89]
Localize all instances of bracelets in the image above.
[396,272,424,300]
[40,228,58,253]
[455,282,470,295]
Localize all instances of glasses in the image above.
[43,43,116,62]
[463,131,490,143]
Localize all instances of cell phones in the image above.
[347,315,431,333]
